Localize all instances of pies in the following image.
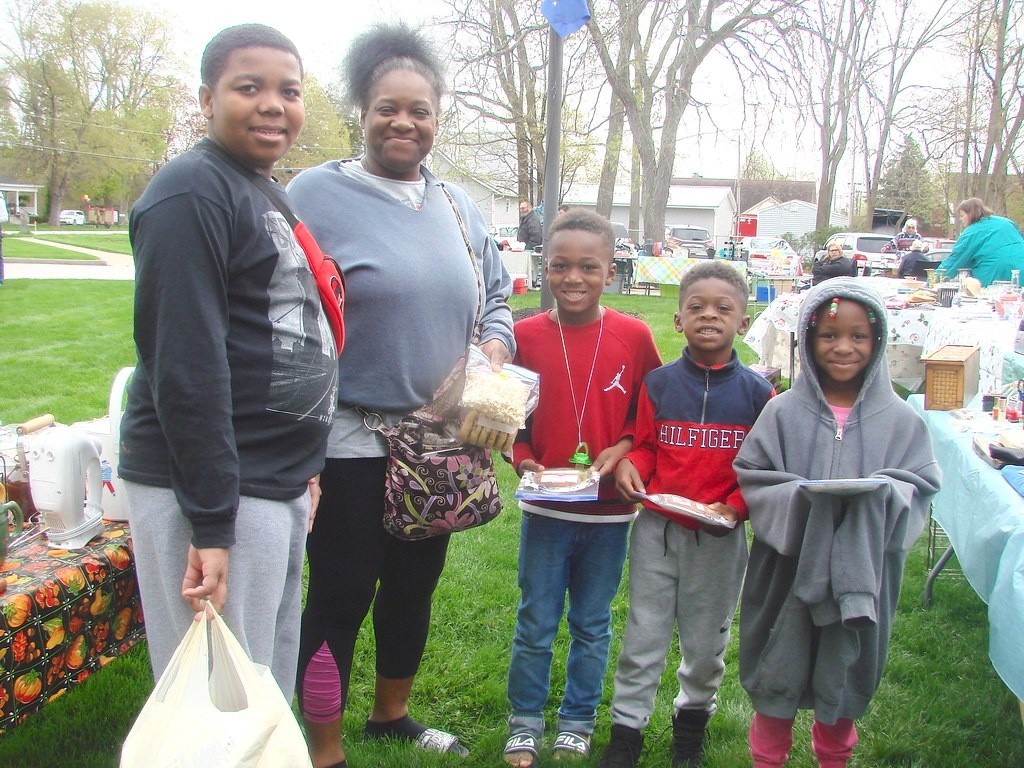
[458,410,518,452]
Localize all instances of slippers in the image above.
[502,733,541,768]
[551,731,591,766]
[413,728,469,760]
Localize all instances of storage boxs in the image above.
[602,274,625,294]
[749,363,781,394]
[757,287,774,302]
[612,260,628,273]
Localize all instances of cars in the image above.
[491,224,527,249]
[733,236,799,271]
[923,248,952,261]
[60,210,86,226]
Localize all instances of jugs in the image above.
[643,239,662,256]
[0,501,23,566]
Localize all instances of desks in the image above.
[0,503,147,735]
[498,250,537,289]
[530,253,1024,699]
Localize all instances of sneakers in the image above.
[671,709,709,768]
[598,724,644,768]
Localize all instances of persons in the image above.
[880,218,929,264]
[557,204,570,217]
[615,260,779,768]
[492,205,667,768]
[287,22,517,767]
[116,24,347,768]
[516,201,543,290]
[0,189,9,285]
[896,239,932,283]
[916,197,1024,292]
[729,276,944,767]
[811,241,853,286]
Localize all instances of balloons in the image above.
[82,194,91,204]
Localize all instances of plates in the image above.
[796,478,888,496]
[523,468,601,492]
[646,494,736,529]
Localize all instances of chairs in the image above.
[911,260,941,281]
[851,260,858,277]
[862,261,872,275]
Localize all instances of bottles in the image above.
[952,268,983,322]
[991,390,1024,423]
[497,240,511,251]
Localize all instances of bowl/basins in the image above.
[903,282,923,290]
[535,246,542,252]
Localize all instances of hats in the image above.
[910,240,925,250]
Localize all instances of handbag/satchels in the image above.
[197,137,345,357]
[362,411,503,541]
[118,599,314,768]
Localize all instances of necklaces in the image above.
[555,304,604,472]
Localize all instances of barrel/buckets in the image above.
[6,464,39,522]
[757,287,775,302]
[85,366,136,523]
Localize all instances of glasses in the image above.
[828,249,839,251]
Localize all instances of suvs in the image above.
[813,232,900,275]
[921,237,956,261]
[610,222,632,254]
[666,225,714,258]
